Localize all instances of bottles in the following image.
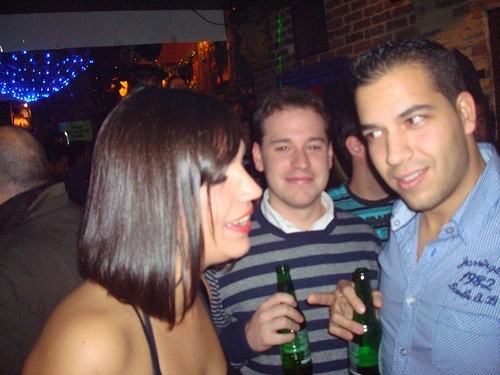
[346,268,384,375]
[275,264,313,375]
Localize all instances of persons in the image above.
[0,124,86,375]
[327,102,397,244]
[471,93,489,141]
[0,61,272,198]
[349,37,500,375]
[203,87,382,375]
[21,86,263,375]
